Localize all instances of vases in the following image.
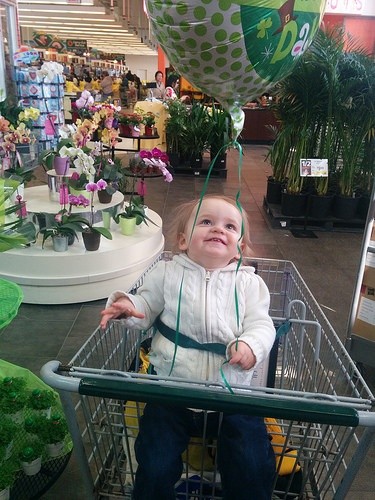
[54,155,70,174]
[69,184,92,204]
[120,216,136,235]
[119,124,129,136]
[145,126,152,136]
[52,234,68,252]
[83,228,100,251]
[98,189,112,202]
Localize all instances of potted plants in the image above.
[167,99,228,170]
[267,24,375,216]
[0,376,70,500]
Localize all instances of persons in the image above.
[122,70,141,99]
[69,72,76,81]
[163,74,190,104]
[101,71,113,98]
[151,71,165,98]
[101,195,277,500]
[85,71,92,83]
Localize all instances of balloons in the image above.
[143,0,326,144]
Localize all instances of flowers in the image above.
[0,86,172,239]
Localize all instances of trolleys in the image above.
[35,255,375,500]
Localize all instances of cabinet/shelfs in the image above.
[17,72,66,155]
[33,50,128,80]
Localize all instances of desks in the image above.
[237,108,279,143]
